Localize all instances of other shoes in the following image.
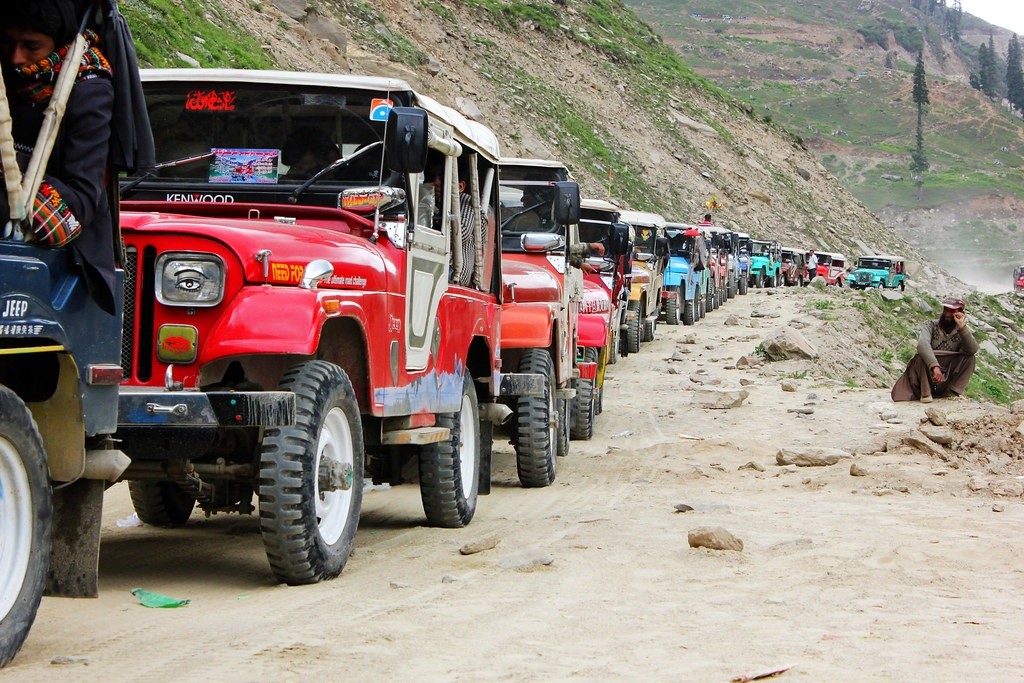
[920,393,933,403]
[941,389,964,400]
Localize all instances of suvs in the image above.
[115,67,502,585]
[567,199,635,442]
[616,210,909,358]
[494,157,583,490]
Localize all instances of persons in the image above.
[908,297,979,403]
[281,126,342,182]
[896,261,902,274]
[0,0,117,246]
[431,167,490,287]
[807,250,819,281]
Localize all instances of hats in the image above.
[941,297,965,310]
